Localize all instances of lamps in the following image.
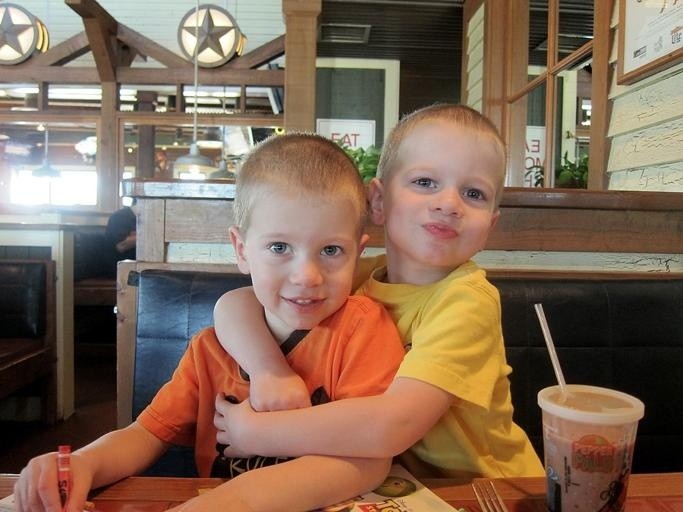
[175,1,215,171]
[33,122,61,177]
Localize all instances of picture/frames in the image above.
[617,0,682,86]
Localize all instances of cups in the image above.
[537,383,644,512]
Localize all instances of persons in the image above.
[13,131,405,512]
[212,102,544,479]
[97,195,136,281]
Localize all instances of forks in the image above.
[468,477,510,512]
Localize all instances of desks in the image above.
[0,471,681,512]
